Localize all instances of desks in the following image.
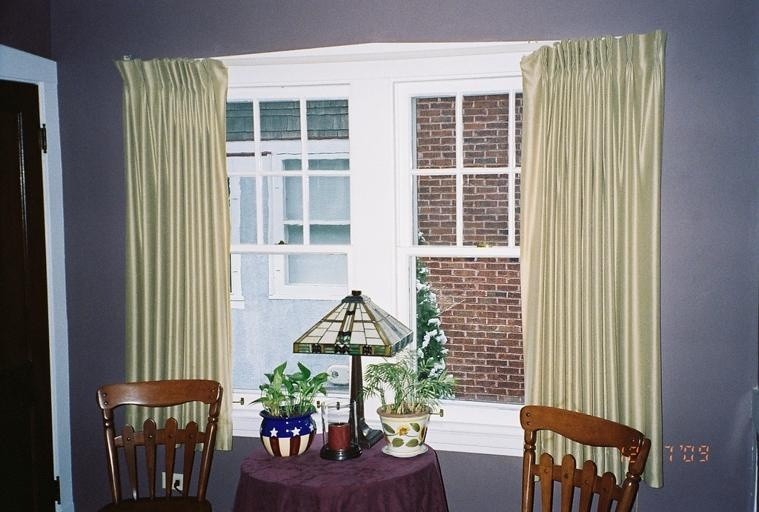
[232,432,448,511]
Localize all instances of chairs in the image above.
[519,405,653,512]
[96,379,223,512]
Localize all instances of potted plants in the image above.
[248,361,331,458]
[357,350,455,459]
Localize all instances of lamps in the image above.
[292,290,415,449]
